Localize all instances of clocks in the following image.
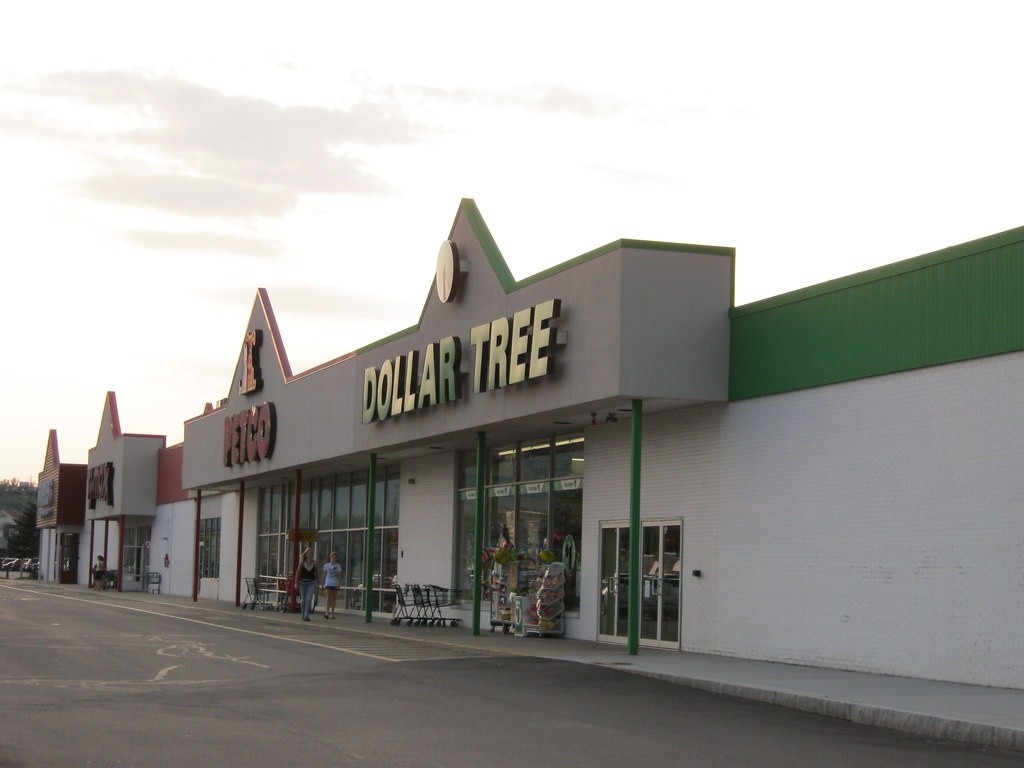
[436,240,460,304]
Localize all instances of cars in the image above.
[0,558,69,574]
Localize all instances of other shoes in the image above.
[324,614,329,619]
[301,616,310,621]
[330,615,335,619]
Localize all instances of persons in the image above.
[11,556,33,569]
[94,555,106,591]
[322,552,342,619]
[294,547,321,621]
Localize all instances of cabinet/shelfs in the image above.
[490,561,564,638]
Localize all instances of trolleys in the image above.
[242,570,317,613]
[390,582,464,628]
[143,572,161,595]
